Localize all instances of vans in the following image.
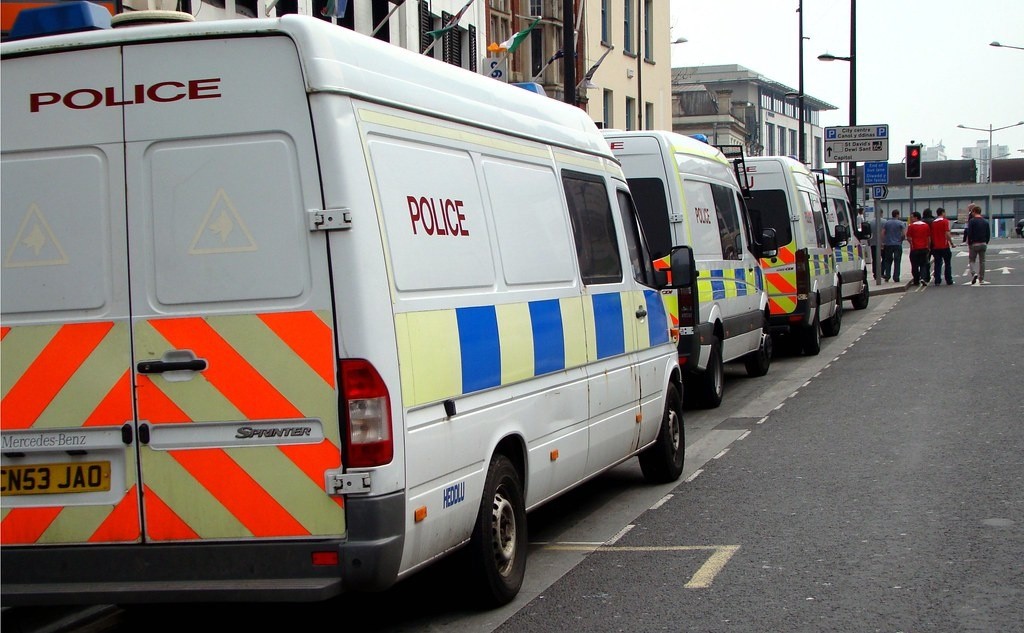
[725,156,844,357]
[0,9,700,612]
[600,127,779,410]
[812,168,873,310]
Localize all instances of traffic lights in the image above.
[906,145,922,178]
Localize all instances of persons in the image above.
[931,208,956,286]
[857,208,868,245]
[867,208,887,280]
[906,211,931,286]
[921,208,935,224]
[960,203,991,284]
[881,209,905,282]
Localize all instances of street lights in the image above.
[817,0,858,205]
[957,121,1024,238]
[784,0,810,165]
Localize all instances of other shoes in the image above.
[980,280,991,285]
[951,282,955,284]
[909,281,914,285]
[921,279,928,286]
[972,274,978,284]
[935,284,940,286]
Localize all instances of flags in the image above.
[548,46,577,65]
[582,50,611,89]
[426,0,474,41]
[488,18,540,53]
